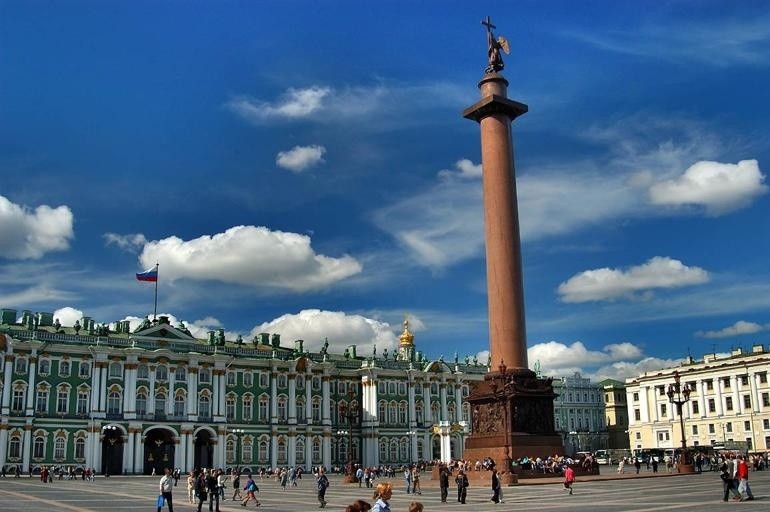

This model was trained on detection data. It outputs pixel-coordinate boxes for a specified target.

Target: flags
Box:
[136,261,159,282]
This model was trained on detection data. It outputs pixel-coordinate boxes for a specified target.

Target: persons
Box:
[344,483,424,512]
[404,456,501,504]
[581,450,770,474]
[260,465,304,491]
[152,466,260,511]
[720,452,754,501]
[3,464,96,484]
[565,464,576,495]
[512,453,575,474]
[316,471,329,509]
[313,464,396,488]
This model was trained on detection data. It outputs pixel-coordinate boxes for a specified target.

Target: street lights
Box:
[404,430,418,467]
[334,428,350,470]
[719,422,729,450]
[98,422,121,478]
[624,429,634,454]
[745,408,759,455]
[568,430,580,458]
[666,368,695,475]
[229,428,248,470]
[488,356,523,488]
[338,385,365,485]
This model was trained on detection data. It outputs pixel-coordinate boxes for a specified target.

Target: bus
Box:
[577,446,727,465]
